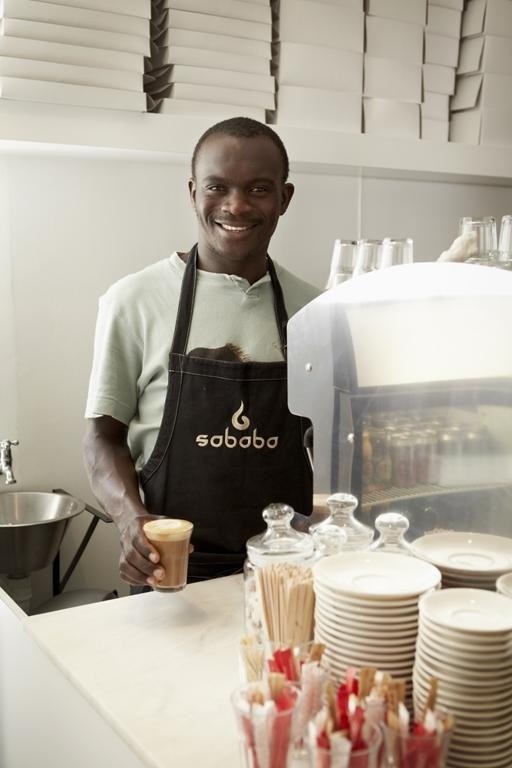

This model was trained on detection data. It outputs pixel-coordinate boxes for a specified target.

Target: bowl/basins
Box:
[0,492,87,577]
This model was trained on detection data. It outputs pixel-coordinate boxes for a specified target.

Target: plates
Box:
[313,533,512,742]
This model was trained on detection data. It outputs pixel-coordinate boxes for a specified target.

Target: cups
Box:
[326,235,413,294]
[141,517,194,594]
[458,213,512,272]
[232,677,452,767]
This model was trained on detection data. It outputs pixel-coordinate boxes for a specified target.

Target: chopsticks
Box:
[253,562,317,653]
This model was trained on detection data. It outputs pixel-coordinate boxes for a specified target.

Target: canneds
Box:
[360,405,493,493]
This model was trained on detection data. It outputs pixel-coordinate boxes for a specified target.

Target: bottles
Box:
[244,491,419,641]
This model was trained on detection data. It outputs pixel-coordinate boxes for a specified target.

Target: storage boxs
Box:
[1,0,511,151]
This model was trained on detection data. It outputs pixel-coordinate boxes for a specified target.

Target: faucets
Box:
[0,439,20,485]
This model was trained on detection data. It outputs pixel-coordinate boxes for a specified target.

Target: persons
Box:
[85,117,363,595]
